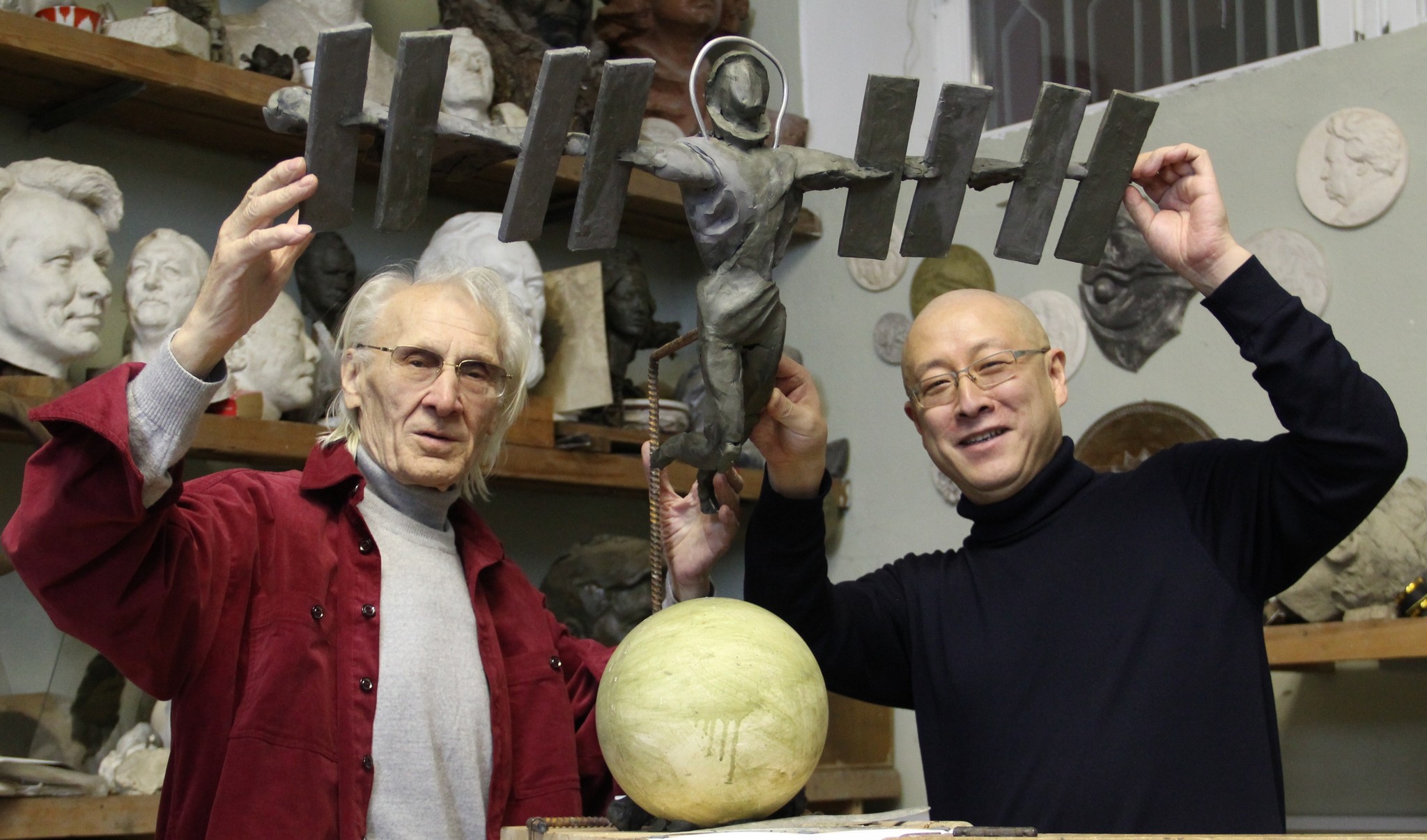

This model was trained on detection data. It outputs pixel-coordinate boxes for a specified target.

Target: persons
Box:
[597,0,811,146]
[221,289,318,425]
[267,49,1096,516]
[115,227,211,371]
[1,156,123,384]
[4,156,746,840]
[742,146,1410,834]
[538,533,668,648]
[293,229,358,341]
[1275,478,1427,625]
[578,268,680,428]
[441,26,495,126]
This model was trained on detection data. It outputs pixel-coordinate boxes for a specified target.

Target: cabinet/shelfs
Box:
[0,6,901,840]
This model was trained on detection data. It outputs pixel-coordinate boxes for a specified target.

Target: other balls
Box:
[595,596,831,827]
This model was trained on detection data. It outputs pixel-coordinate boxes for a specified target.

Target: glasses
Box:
[354,342,513,397]
[911,347,1047,407]
